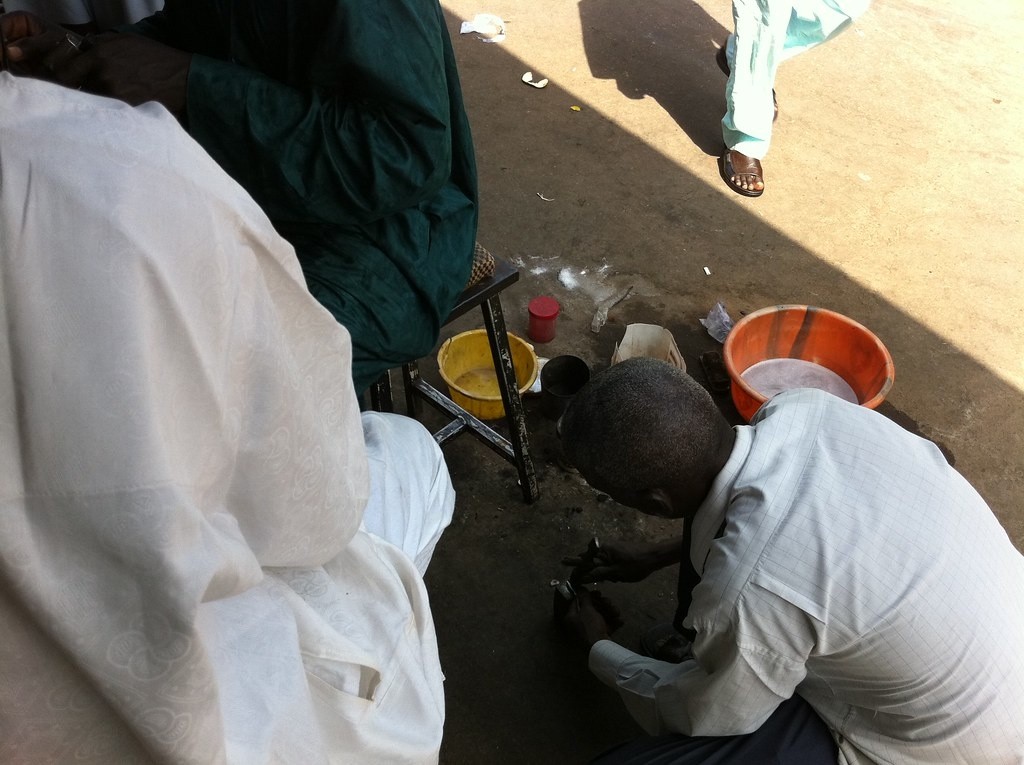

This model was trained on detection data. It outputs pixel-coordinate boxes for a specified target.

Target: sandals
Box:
[723,140,764,197]
[716,36,778,124]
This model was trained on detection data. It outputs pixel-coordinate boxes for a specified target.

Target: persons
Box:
[563,356,1024,765]
[0,64,458,763]
[716,1,871,197]
[0,0,479,397]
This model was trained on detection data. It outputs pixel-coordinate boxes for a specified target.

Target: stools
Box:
[362,242,541,502]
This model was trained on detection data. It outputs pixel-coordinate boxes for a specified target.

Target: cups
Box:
[541,354,590,422]
[527,298,559,344]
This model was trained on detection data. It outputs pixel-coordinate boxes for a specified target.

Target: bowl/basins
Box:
[723,303,895,424]
[436,328,538,420]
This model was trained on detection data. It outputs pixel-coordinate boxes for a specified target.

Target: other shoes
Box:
[642,623,695,664]
